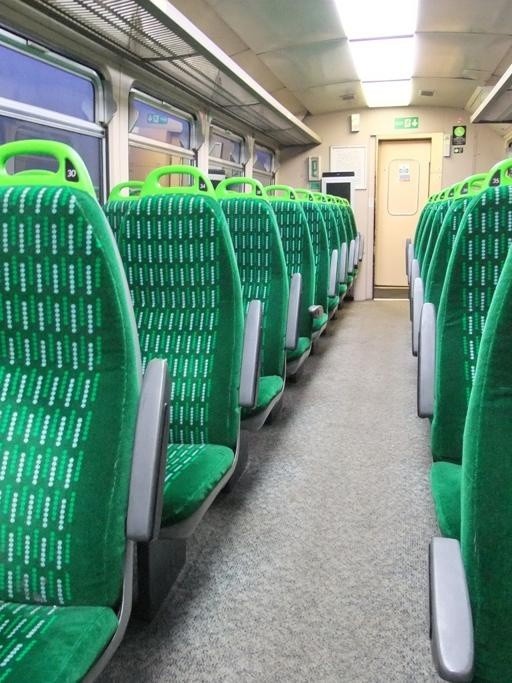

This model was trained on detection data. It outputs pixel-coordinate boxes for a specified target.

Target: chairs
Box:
[403,157,511,683]
[0,138,363,681]
